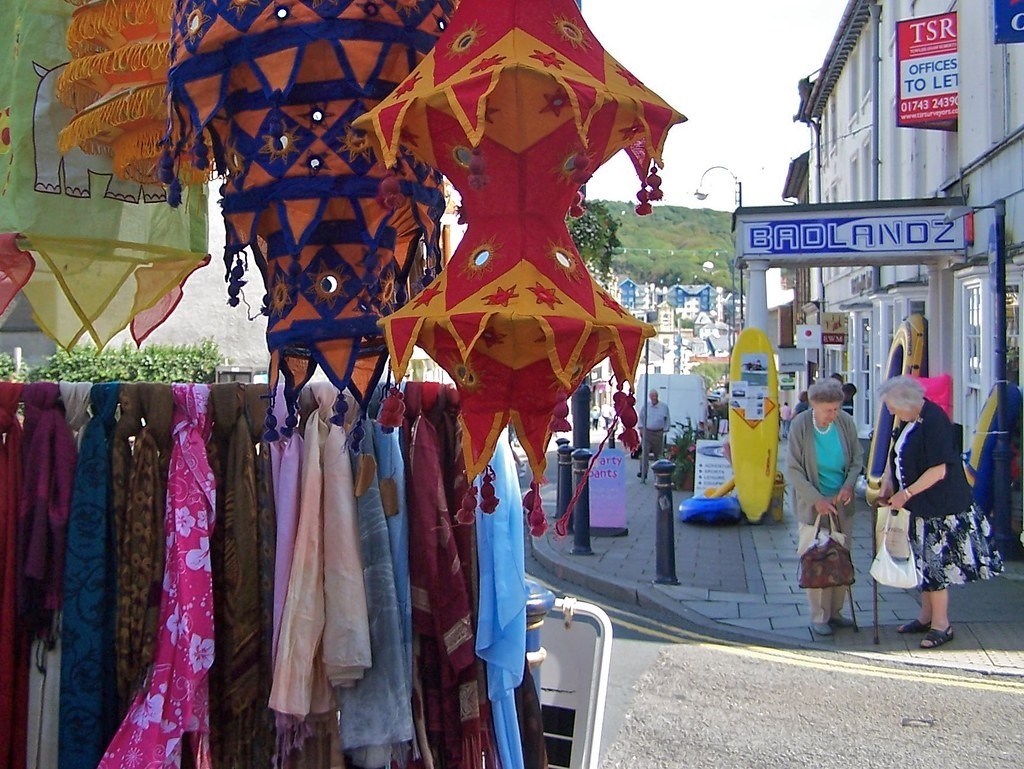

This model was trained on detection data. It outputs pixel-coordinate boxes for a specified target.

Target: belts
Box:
[646,428,663,432]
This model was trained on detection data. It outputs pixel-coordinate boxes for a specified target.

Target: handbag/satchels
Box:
[876,507,910,558]
[798,512,855,588]
[796,513,845,555]
[869,505,918,589]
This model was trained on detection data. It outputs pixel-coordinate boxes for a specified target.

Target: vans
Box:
[635,373,709,450]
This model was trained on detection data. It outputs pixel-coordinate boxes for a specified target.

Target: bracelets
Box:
[905,489,912,499]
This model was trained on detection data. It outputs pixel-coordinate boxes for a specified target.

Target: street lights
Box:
[702,246,735,348]
[943,198,1024,561]
[693,166,745,335]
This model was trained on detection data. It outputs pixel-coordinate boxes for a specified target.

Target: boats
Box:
[677,487,742,524]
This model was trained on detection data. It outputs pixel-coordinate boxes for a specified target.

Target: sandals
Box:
[920,626,953,648]
[898,618,931,633]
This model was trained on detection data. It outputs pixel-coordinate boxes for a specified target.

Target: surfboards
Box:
[726,327,780,527]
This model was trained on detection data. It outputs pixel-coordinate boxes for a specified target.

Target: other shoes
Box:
[812,623,833,636]
[833,616,852,625]
[637,473,647,478]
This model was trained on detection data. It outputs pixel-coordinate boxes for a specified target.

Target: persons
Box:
[786,379,863,634]
[715,383,729,431]
[778,403,791,441]
[637,389,670,478]
[877,376,1004,648]
[792,391,809,417]
[830,373,857,416]
[590,402,613,429]
